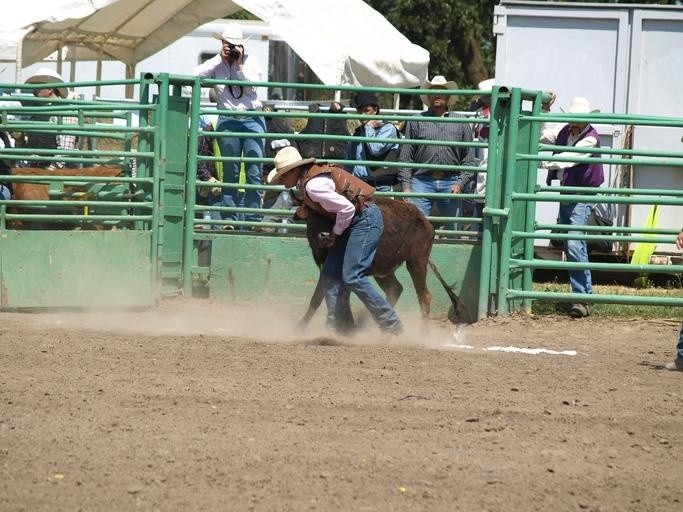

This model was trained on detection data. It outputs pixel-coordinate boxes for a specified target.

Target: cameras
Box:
[229,43,240,61]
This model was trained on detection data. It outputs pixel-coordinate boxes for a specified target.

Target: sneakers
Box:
[569,299,591,318]
[224,224,235,231]
[251,224,264,232]
[666,361,682,372]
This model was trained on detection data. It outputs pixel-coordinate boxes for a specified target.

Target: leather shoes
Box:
[380,322,403,344]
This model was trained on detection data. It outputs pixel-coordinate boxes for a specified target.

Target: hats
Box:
[349,93,379,108]
[421,73,459,107]
[473,77,496,101]
[267,146,316,184]
[559,96,601,114]
[22,66,70,99]
[210,24,253,46]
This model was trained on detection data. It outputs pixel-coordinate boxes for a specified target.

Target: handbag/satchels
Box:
[587,214,612,254]
[365,121,404,185]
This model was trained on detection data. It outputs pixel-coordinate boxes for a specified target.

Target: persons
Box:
[539,96,604,316]
[266,146,406,340]
[666,227,683,371]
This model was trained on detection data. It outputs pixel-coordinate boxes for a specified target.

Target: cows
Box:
[289,188,472,336]
[7,165,132,230]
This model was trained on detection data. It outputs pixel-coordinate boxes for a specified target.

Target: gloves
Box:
[200,186,209,198]
[208,177,222,195]
